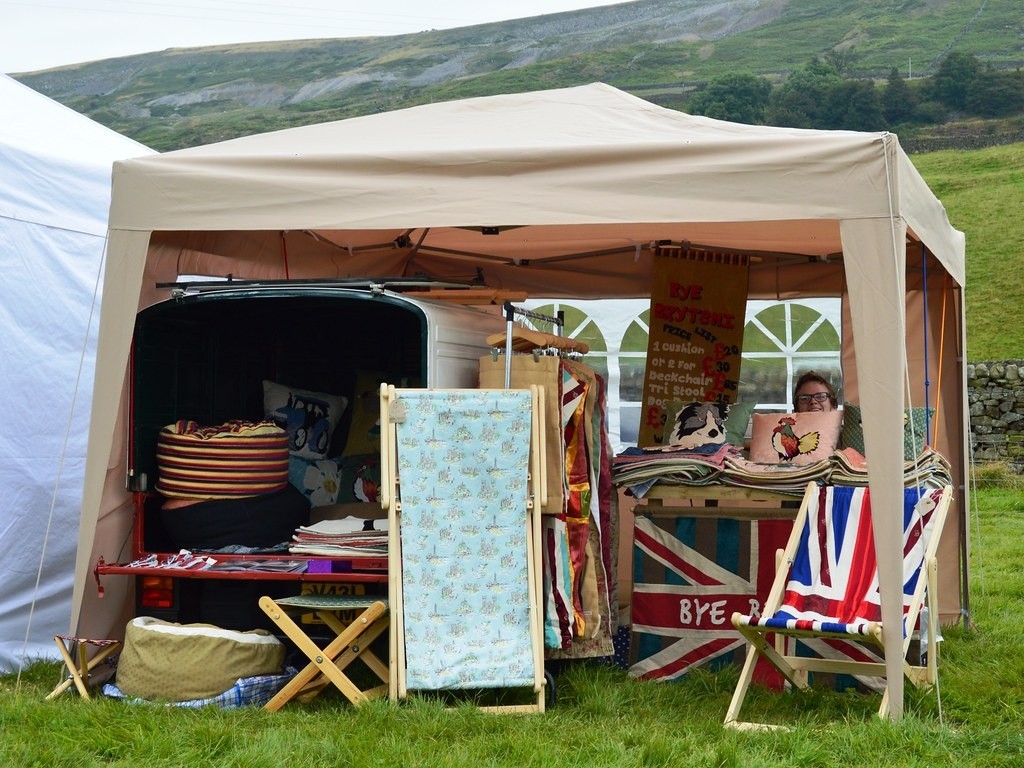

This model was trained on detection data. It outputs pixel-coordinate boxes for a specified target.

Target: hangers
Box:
[487,307,589,362]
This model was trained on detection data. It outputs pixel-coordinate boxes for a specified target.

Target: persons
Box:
[792,370,839,412]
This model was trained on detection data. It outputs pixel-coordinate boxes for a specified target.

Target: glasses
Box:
[795,392,831,404]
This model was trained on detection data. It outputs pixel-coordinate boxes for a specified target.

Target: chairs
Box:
[722,481,955,733]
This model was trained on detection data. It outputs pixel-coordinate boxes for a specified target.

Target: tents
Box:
[0,70,159,676]
[63,81,971,728]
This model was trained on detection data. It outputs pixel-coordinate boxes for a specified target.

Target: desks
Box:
[623,483,804,678]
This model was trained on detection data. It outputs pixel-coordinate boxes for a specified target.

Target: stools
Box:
[258,594,389,714]
[45,633,121,703]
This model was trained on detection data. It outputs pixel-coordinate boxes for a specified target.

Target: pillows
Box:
[842,401,936,461]
[749,409,845,466]
[662,399,759,454]
[262,379,386,505]
